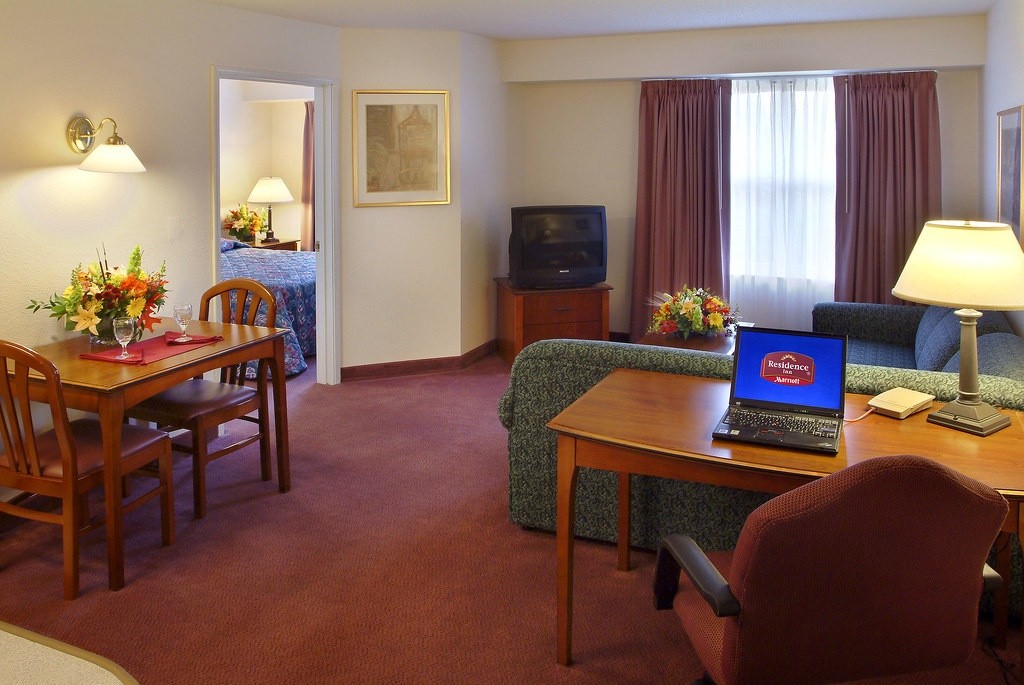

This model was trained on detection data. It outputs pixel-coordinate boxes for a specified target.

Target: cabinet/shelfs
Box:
[493,277,615,367]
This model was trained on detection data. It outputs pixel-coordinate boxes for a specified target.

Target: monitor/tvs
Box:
[508,205,607,287]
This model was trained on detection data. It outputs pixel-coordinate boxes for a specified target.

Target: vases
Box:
[678,330,707,338]
[89,315,138,348]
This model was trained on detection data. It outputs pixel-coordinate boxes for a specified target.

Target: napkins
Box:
[78,331,225,367]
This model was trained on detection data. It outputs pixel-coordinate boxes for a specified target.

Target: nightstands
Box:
[244,238,301,251]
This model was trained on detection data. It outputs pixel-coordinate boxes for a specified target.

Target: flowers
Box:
[643,280,743,339]
[25,241,172,340]
[223,203,268,236]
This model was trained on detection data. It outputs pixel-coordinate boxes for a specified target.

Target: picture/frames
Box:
[996,104,1024,253]
[351,88,452,208]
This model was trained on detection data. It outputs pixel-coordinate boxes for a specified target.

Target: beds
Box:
[221,237,317,380]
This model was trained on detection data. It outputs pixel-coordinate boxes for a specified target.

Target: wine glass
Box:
[113,317,135,359]
[174,304,193,342]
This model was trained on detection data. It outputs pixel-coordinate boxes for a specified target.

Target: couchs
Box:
[812,302,1024,382]
[498,338,1024,627]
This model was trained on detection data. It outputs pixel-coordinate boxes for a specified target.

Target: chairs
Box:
[0,339,176,600]
[649,455,1011,685]
[122,277,278,518]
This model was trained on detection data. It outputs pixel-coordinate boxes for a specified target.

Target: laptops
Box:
[712,325,848,454]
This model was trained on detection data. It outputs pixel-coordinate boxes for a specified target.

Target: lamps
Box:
[892,220,1024,438]
[247,176,294,243]
[65,116,147,174]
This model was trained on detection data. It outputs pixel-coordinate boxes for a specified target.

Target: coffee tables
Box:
[636,322,755,356]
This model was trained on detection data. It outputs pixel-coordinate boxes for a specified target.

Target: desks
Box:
[547,368,1024,668]
[0,316,293,590]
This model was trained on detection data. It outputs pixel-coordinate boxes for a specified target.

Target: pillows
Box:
[220,238,251,253]
